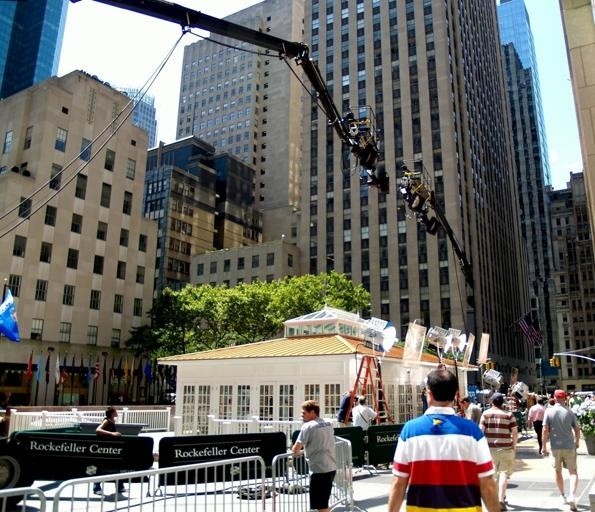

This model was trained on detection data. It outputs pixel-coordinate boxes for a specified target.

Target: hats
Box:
[553,390,566,400]
[490,392,504,406]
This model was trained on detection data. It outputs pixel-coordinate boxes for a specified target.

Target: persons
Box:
[335,388,358,427]
[387,368,503,512]
[93,406,131,496]
[460,388,595,511]
[292,399,336,512]
[351,396,377,452]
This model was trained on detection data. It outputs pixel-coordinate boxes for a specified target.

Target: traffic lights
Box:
[487,362,492,370]
[550,359,554,367]
[555,359,560,366]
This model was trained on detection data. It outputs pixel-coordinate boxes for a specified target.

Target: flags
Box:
[518,312,544,347]
[0,288,21,342]
[24,351,176,386]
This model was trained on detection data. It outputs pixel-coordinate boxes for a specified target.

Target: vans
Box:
[570,391,594,401]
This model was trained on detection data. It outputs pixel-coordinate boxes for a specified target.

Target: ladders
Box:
[344,355,395,426]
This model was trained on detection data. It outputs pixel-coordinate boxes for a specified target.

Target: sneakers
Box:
[499,502,507,511]
[559,494,578,511]
